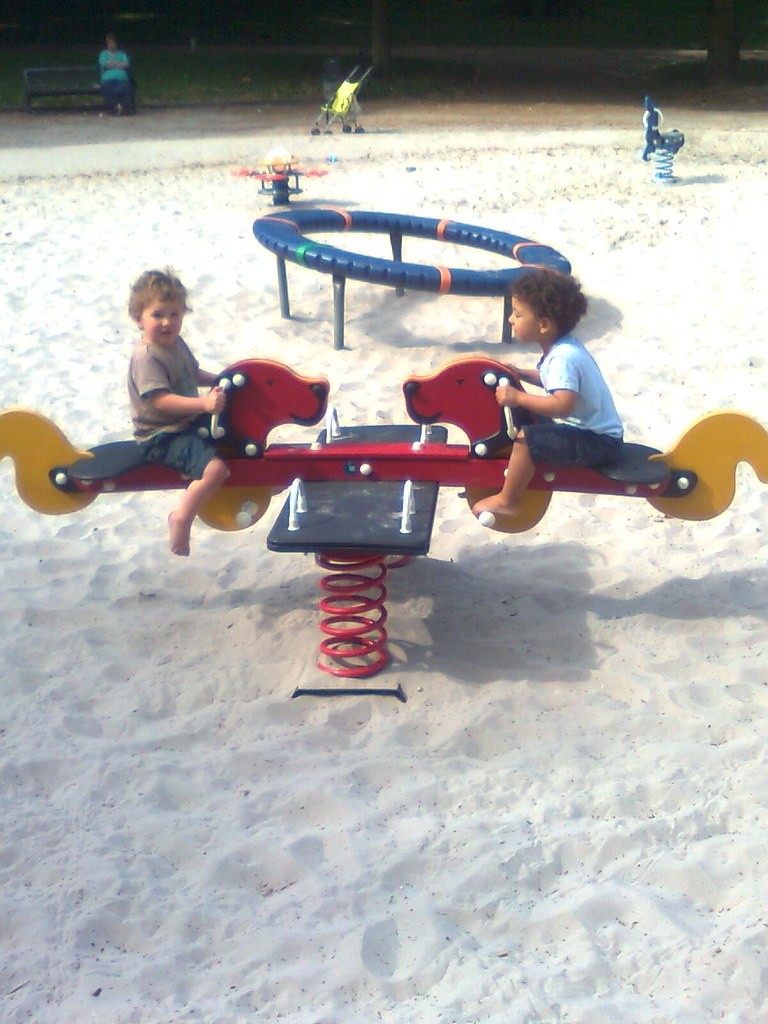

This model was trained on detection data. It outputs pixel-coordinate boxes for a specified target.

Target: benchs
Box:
[23,66,138,114]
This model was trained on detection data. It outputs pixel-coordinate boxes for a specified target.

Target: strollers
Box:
[311,65,370,136]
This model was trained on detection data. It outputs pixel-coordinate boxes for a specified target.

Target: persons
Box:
[98,32,132,114]
[472,269,625,520]
[128,269,230,556]
